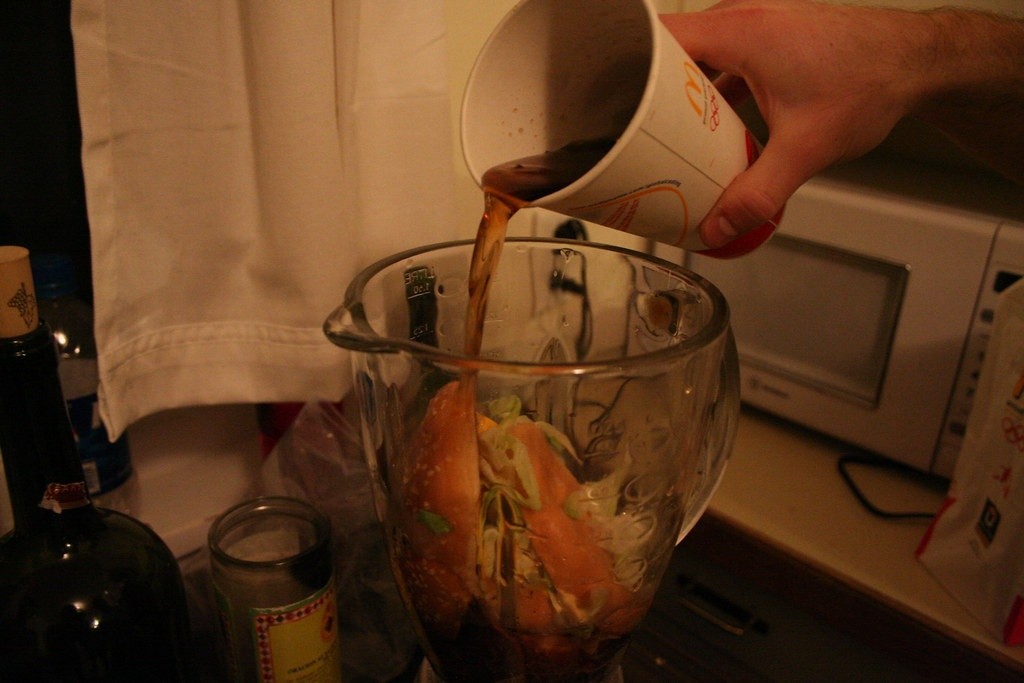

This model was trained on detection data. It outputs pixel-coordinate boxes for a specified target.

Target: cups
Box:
[459,0,791,260]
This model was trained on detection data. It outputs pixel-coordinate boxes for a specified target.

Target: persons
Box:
[657,0,1024,248]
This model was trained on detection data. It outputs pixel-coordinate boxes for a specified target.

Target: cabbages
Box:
[417,396,657,623]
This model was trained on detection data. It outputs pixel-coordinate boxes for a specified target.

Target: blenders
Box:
[320,237,741,683]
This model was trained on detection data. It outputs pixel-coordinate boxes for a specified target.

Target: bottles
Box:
[0,243,203,683]
[29,267,143,517]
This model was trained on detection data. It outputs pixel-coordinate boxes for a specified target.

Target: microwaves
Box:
[642,176,1023,481]
